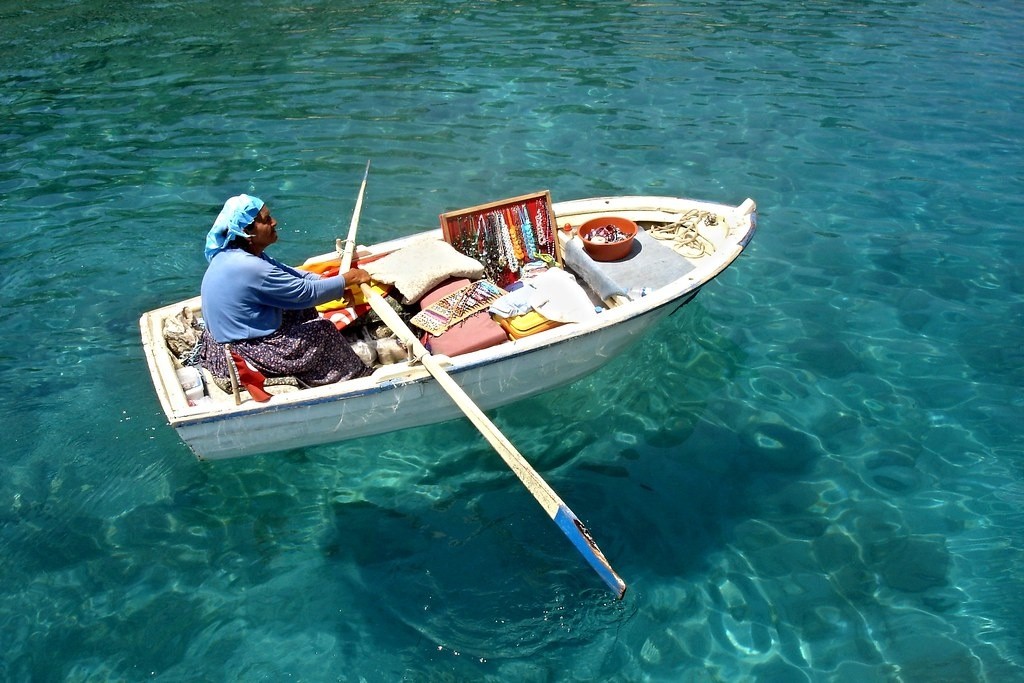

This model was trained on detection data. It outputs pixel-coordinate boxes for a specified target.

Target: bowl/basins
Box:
[578,216,638,260]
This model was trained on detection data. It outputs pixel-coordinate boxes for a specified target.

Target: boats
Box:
[140,195,755,462]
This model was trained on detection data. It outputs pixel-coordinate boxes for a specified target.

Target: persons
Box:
[196,193,377,390]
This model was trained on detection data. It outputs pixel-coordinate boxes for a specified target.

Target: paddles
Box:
[337,158,372,277]
[358,278,631,601]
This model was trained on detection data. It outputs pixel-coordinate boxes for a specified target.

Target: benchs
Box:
[199,365,297,407]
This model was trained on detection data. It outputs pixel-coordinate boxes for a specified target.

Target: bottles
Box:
[563,223,573,239]
